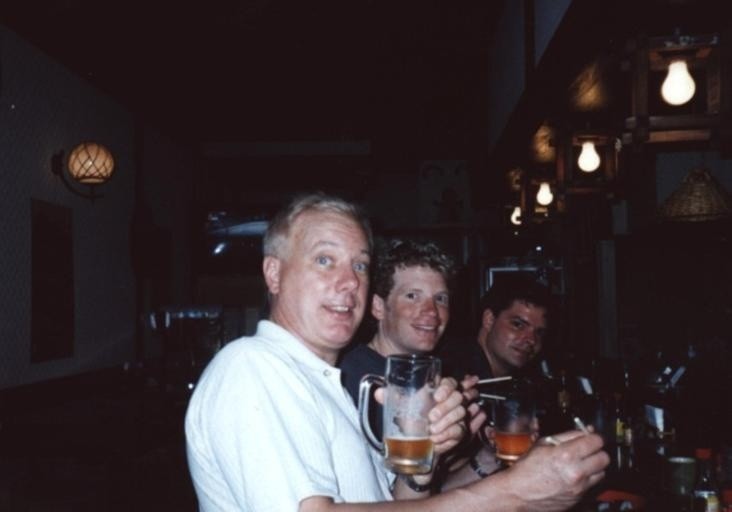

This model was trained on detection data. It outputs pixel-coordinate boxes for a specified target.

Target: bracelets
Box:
[400,472,431,492]
[469,458,488,479]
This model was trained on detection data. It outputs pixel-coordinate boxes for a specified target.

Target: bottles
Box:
[686,447,723,512]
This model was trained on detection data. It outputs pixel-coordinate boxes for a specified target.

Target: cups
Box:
[666,457,697,496]
[356,352,443,475]
[476,377,543,462]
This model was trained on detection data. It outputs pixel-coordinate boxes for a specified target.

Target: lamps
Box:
[577,139,602,172]
[656,49,696,107]
[535,181,554,206]
[52,139,116,206]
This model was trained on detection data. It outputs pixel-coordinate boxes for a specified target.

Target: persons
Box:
[340,235,541,494]
[183,192,612,512]
[447,270,551,451]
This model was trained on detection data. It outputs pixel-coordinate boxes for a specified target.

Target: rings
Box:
[458,422,467,436]
